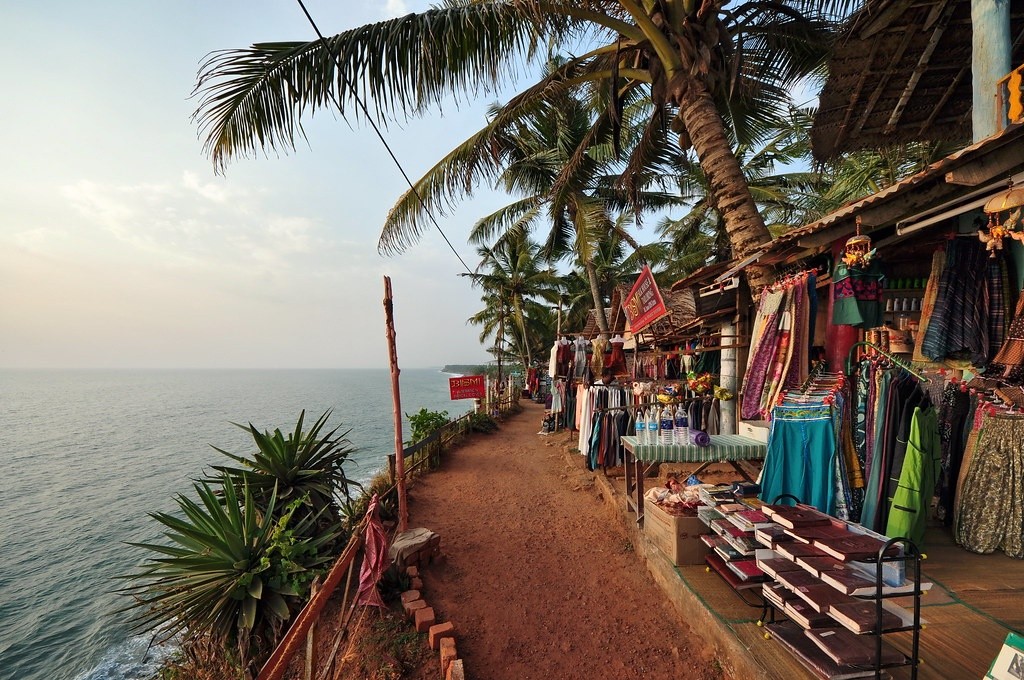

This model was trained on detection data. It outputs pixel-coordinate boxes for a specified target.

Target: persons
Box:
[590,335,608,374]
[594,368,616,384]
[608,334,627,374]
[573,336,590,377]
[555,337,572,376]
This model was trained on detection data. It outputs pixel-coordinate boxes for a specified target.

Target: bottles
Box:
[660,407,673,445]
[635,412,645,444]
[648,412,658,445]
[675,407,689,446]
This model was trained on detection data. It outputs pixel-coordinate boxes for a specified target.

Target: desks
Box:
[620,433,769,528]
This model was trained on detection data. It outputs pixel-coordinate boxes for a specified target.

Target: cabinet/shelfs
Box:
[696,479,801,627]
[754,495,929,680]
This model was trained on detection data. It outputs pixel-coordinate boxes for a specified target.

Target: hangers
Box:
[595,394,720,416]
[761,259,819,290]
[778,342,1024,414]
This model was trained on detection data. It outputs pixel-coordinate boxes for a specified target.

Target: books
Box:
[697,481,906,680]
